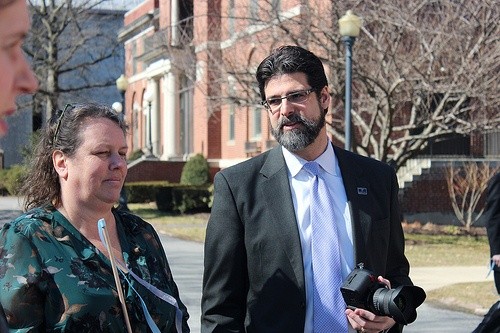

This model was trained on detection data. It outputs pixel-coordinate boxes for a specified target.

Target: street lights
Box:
[144,90,156,159]
[337,9,363,152]
[116,74,129,159]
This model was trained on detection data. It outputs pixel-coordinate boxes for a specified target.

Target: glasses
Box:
[52,103,84,151]
[260,87,320,111]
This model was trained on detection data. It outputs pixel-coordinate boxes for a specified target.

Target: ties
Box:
[302,162,349,333]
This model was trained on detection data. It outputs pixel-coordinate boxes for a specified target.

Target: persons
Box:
[199,45,415,333]
[0,102,191,333]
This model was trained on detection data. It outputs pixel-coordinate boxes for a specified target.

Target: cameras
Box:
[340,262,426,326]
